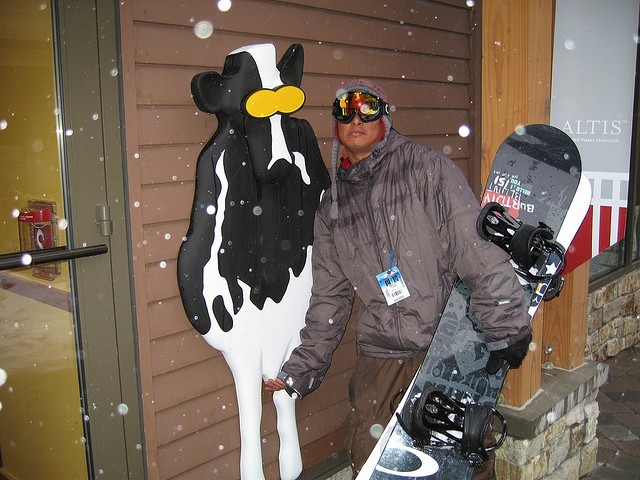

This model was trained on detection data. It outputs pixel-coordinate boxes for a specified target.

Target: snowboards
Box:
[368,123,582,480]
[353,171,592,480]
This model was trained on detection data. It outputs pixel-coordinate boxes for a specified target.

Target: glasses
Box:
[331,91,388,124]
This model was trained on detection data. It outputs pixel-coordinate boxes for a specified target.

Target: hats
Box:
[328,77,394,220]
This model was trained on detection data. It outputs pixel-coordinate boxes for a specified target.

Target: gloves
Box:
[484,328,533,376]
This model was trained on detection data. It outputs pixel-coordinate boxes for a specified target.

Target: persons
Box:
[265,78,534,480]
